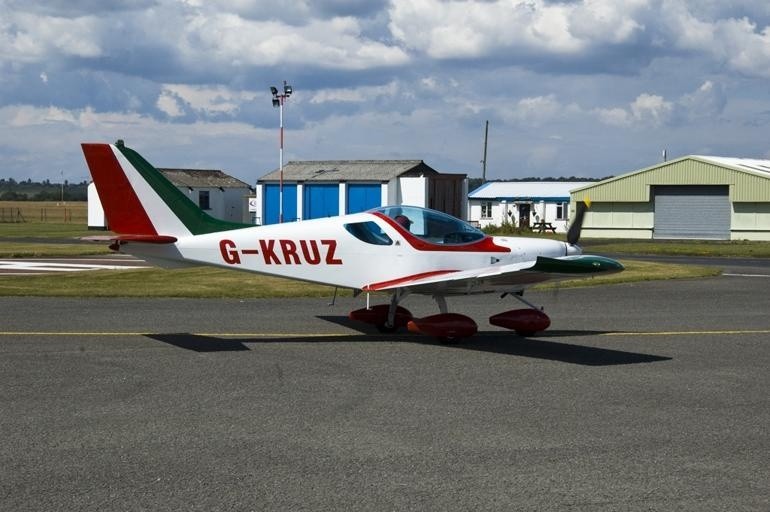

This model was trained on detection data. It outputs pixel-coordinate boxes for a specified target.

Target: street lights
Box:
[268,81,293,225]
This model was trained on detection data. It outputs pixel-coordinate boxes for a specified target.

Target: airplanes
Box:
[80,135,626,344]
